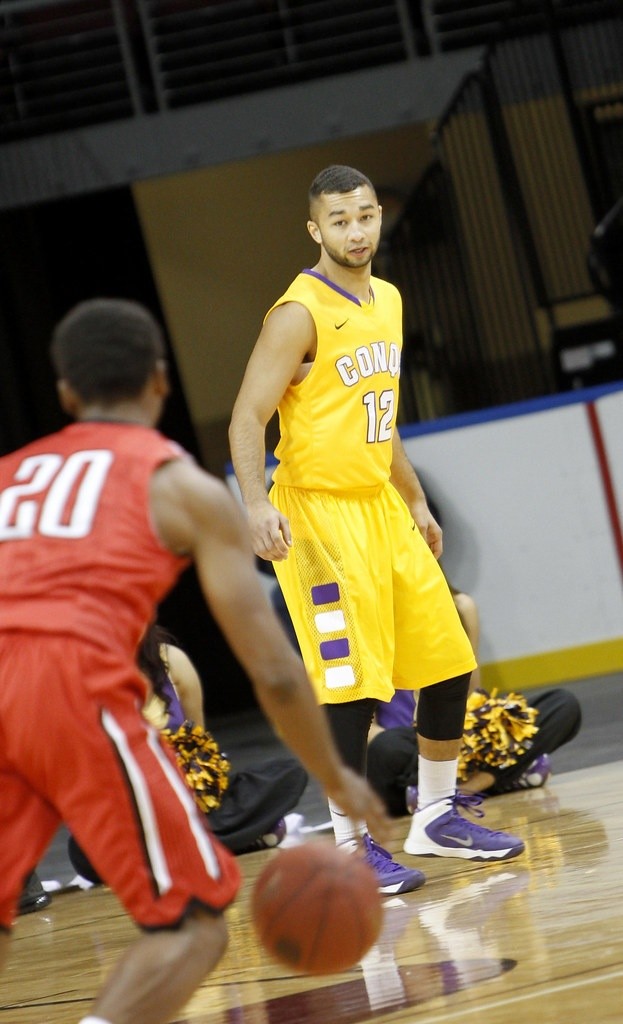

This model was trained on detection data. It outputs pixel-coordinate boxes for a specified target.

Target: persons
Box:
[18,619,310,919]
[227,166,525,895]
[254,446,581,820]
[0,302,399,1024]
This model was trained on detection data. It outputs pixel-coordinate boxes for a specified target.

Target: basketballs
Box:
[250,841,383,973]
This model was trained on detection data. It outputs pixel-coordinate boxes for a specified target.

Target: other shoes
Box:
[19,892,50,917]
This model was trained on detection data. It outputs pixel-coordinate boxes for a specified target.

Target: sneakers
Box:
[487,753,551,795]
[403,789,527,862]
[236,818,287,854]
[406,786,418,814]
[342,833,425,895]
